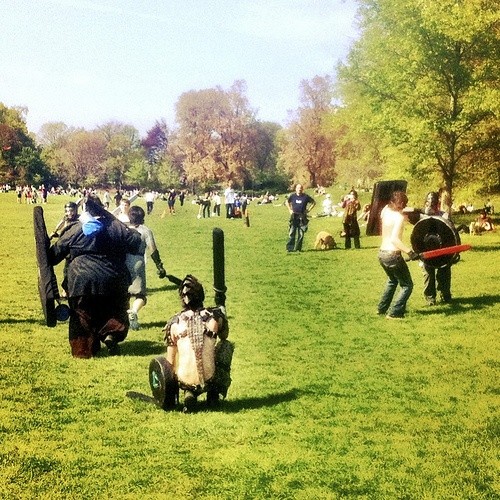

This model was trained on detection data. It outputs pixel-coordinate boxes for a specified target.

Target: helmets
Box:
[64,201,77,208]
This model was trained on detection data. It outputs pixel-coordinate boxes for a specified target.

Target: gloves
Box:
[150,249,166,279]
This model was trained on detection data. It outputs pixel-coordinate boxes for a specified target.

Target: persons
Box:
[341,189,361,250]
[0,184,376,227]
[409,199,494,236]
[49,201,83,241]
[375,191,420,320]
[163,274,234,411]
[285,184,316,253]
[49,194,142,358]
[125,205,166,331]
[406,191,451,305]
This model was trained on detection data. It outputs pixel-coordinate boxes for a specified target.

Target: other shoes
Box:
[441,295,451,302]
[184,392,197,412]
[386,313,406,320]
[428,299,436,306]
[104,335,120,349]
[128,311,139,331]
[376,309,387,316]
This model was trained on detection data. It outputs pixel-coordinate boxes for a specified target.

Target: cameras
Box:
[348,195,354,199]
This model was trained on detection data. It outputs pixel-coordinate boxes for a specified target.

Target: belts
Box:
[380,249,401,255]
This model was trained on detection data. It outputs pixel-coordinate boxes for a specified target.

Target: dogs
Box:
[314,231,336,250]
